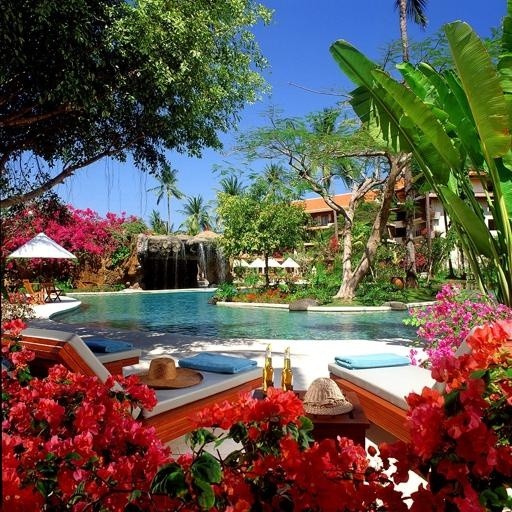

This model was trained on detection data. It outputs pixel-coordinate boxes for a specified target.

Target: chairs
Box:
[327,324,492,452]
[23,278,62,304]
[5,324,264,450]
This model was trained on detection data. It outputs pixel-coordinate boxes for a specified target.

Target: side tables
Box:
[249,388,371,453]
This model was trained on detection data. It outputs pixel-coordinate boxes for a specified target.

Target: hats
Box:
[302,378,353,415]
[124,358,203,389]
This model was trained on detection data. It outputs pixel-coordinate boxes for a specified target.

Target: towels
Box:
[334,351,410,371]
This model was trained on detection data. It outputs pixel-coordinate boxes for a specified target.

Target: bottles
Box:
[281,347,293,393]
[262,344,276,395]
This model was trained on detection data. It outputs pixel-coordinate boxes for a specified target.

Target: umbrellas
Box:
[9,233,76,283]
